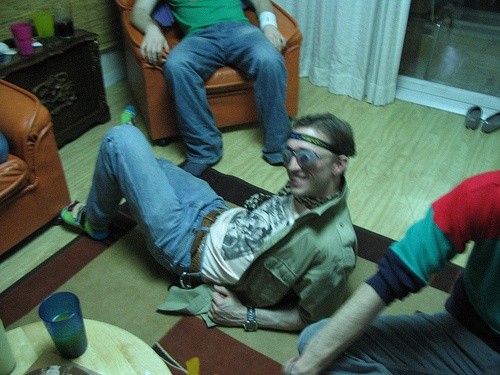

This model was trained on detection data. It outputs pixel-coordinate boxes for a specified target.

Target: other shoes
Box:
[465,104,500,133]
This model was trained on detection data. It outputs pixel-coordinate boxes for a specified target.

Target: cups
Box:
[38,292,88,359]
[32,11,54,39]
[10,22,33,54]
[0,321,16,375]
[55,5,75,39]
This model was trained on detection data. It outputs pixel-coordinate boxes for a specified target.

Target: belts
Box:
[190,209,226,284]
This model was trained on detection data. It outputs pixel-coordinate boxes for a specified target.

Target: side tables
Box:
[0,28,111,150]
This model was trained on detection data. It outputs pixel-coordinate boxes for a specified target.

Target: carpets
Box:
[152,311,286,375]
[0,158,466,375]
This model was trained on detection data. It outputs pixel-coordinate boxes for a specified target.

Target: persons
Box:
[59,102,359,333]
[128,1,295,178]
[281,166,500,375]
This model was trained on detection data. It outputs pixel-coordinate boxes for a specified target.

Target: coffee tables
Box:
[5,318,171,375]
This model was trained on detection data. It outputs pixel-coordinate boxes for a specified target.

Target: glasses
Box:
[282,147,338,167]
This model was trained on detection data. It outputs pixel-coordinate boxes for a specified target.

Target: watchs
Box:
[242,305,258,332]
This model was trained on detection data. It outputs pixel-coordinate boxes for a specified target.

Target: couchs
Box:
[0,78,72,257]
[115,0,304,147]
